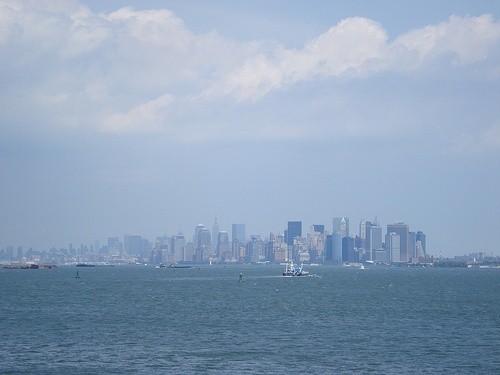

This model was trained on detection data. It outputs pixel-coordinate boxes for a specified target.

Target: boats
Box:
[343,262,365,270]
[282,267,310,276]
[3,261,59,270]
[154,263,193,269]
[75,263,115,267]
[480,265,500,268]
